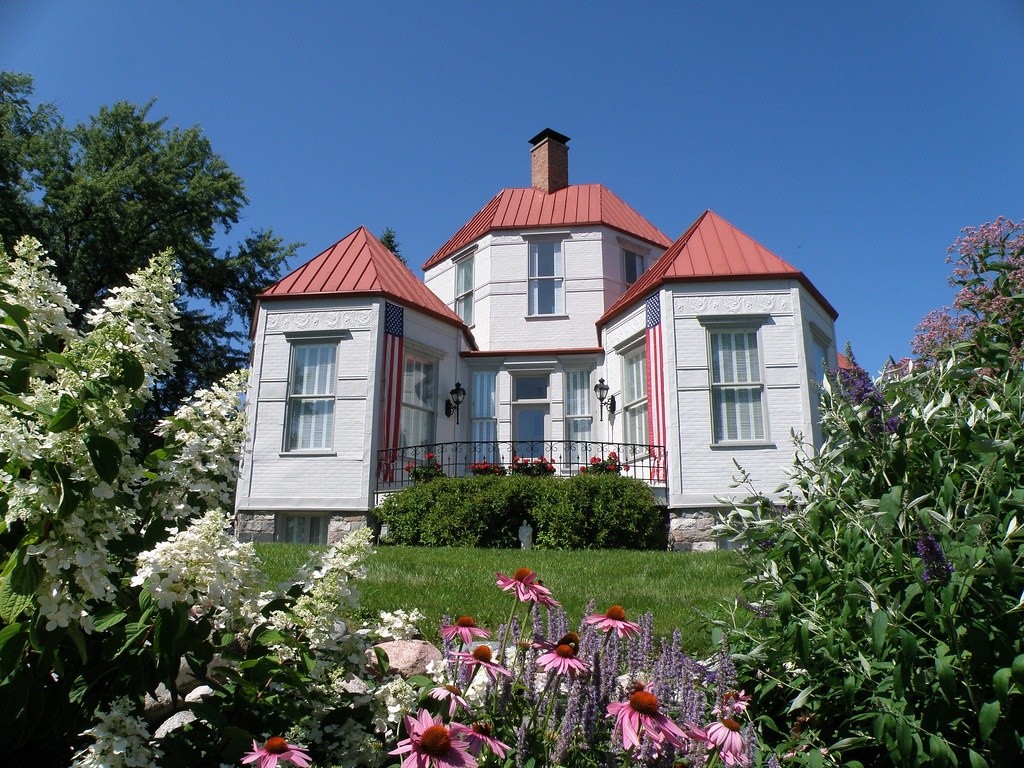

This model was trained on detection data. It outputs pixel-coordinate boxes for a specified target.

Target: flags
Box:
[645,291,667,482]
[382,300,404,482]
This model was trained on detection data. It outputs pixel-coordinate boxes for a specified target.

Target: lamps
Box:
[445,380,466,425]
[593,378,615,422]
